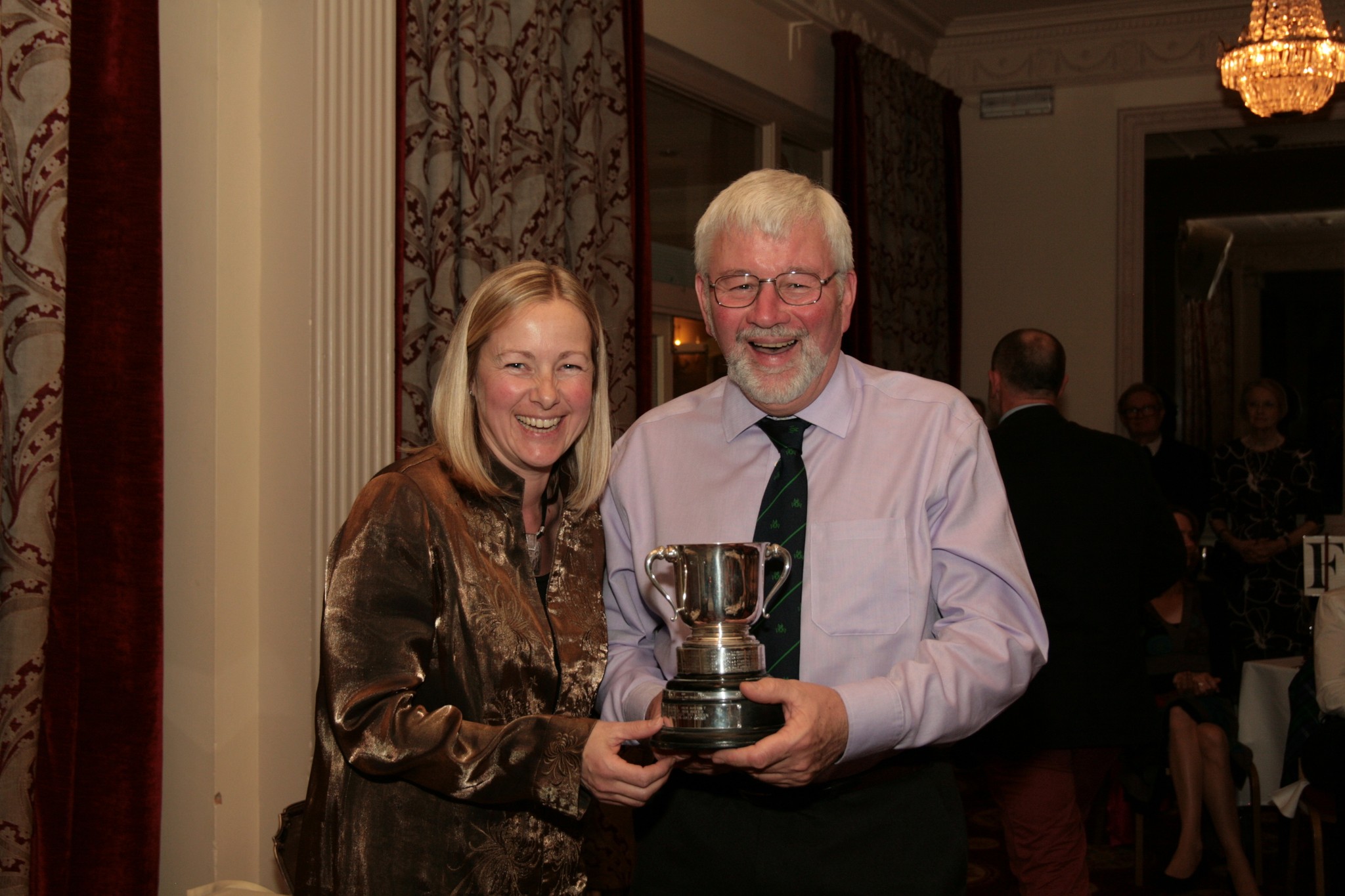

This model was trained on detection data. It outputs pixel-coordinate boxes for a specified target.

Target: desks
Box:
[1232,654,1312,812]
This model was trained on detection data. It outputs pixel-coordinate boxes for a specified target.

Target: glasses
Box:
[708,267,840,308]
[1121,404,1162,418]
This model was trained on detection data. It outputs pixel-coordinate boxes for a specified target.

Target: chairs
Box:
[1285,750,1345,896]
[1113,725,1267,888]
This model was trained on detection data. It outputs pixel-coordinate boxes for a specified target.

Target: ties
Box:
[753,416,813,726]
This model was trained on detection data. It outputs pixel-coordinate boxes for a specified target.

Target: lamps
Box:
[1213,1,1345,118]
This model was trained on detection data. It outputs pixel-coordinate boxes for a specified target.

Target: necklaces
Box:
[523,490,548,573]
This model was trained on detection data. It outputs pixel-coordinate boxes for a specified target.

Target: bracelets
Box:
[1217,527,1229,538]
[1282,533,1293,550]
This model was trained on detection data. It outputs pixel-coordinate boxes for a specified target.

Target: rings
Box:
[1198,682,1203,686]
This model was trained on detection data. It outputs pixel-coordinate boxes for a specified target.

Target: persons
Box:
[602,168,1054,895]
[294,258,679,895]
[987,328,1184,896]
[966,396,998,444]
[1136,507,1266,896]
[1118,381,1208,534]
[1202,376,1329,796]
